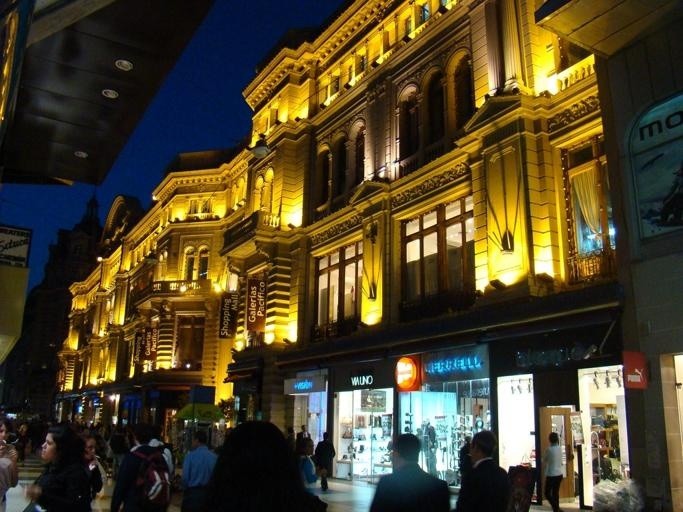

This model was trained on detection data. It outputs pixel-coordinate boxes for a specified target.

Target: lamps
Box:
[590,370,621,389]
[506,377,532,395]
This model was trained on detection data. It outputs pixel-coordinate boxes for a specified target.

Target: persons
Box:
[456,429,510,511]
[369,434,451,512]
[1,416,336,511]
[541,431,567,512]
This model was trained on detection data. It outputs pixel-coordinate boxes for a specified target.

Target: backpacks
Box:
[132,447,172,510]
